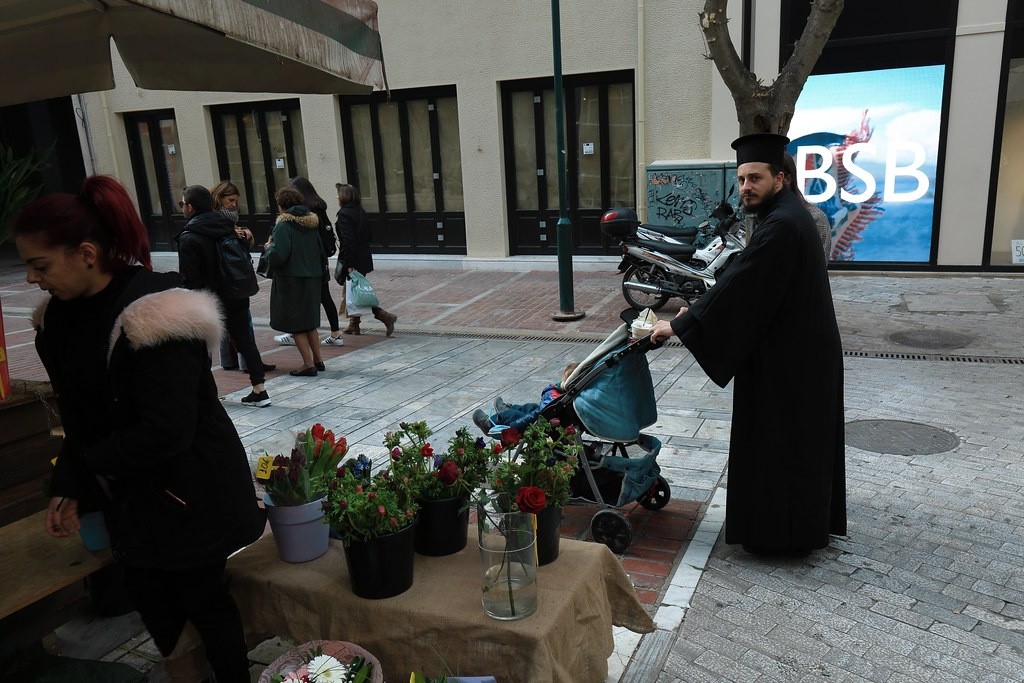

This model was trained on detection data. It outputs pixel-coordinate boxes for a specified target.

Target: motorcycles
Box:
[600,182,747,311]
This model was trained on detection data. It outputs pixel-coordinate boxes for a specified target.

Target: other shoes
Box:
[290,366,317,376]
[244,363,276,373]
[314,362,325,371]
[223,364,240,370]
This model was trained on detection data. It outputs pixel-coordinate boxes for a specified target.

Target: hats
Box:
[731,134,790,168]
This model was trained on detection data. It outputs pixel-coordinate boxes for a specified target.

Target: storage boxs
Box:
[600,208,640,237]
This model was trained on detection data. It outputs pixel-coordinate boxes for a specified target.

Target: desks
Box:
[161,529,655,683]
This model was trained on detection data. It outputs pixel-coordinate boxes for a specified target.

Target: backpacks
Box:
[211,233,259,299]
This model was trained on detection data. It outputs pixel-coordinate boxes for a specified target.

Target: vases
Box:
[477,531,539,621]
[340,524,417,599]
[502,501,562,567]
[259,638,384,683]
[411,490,471,557]
[263,493,333,563]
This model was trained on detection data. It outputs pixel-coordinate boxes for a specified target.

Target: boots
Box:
[374,308,397,336]
[343,316,360,335]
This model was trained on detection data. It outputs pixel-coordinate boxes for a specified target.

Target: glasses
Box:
[179,201,188,208]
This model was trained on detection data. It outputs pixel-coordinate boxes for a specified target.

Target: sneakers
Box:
[494,395,512,413]
[241,390,271,407]
[321,334,344,346]
[473,408,494,437]
[274,332,297,345]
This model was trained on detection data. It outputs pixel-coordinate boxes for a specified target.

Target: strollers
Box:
[485,305,672,552]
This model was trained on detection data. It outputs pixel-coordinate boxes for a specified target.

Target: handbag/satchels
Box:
[345,277,372,319]
[334,262,348,286]
[323,216,337,257]
[257,247,273,279]
[349,270,379,307]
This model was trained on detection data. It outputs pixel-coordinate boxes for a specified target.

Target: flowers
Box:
[263,409,584,540]
[271,646,371,683]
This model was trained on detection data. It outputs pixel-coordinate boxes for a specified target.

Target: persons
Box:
[210,181,276,372]
[274,177,343,346]
[173,185,271,407]
[7,176,269,683]
[264,186,325,377]
[335,185,397,337]
[649,133,846,559]
[473,362,580,440]
[745,153,831,265]
